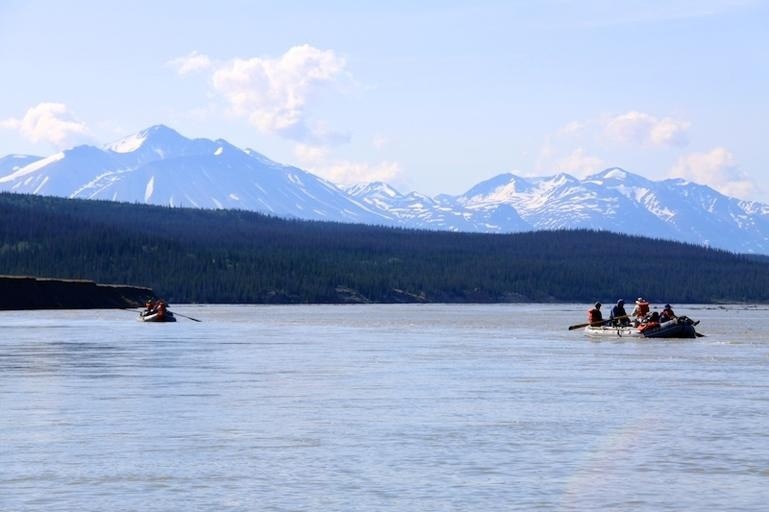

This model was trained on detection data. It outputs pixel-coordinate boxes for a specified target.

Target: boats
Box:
[585,317,697,339]
[135,311,177,322]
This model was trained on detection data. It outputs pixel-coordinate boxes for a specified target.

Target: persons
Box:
[587,301,603,327]
[606,299,633,327]
[631,297,678,328]
[145,296,166,317]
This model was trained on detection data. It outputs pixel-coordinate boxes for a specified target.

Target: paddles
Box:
[569,314,632,330]
[172,312,201,322]
